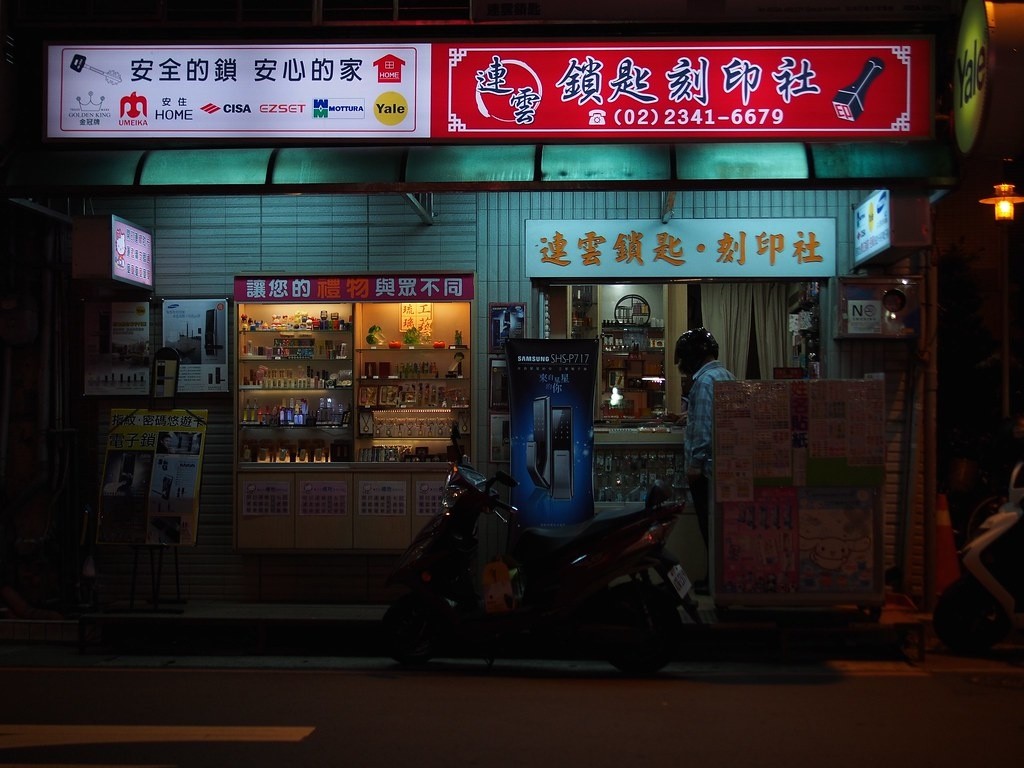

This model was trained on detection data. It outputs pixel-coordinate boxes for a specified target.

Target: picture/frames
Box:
[488,355,510,410]
[487,300,528,357]
[488,413,510,464]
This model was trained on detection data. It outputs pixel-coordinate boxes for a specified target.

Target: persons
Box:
[662,329,737,598]
[159,433,175,454]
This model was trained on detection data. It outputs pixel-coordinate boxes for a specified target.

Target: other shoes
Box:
[694,578,712,595]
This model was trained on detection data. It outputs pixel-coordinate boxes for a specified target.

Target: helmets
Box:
[675,327,719,381]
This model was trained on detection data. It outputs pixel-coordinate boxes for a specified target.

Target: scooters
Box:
[932,459,1024,655]
[380,422,709,678]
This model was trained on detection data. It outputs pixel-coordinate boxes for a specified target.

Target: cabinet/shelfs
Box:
[355,300,474,551]
[234,301,355,548]
